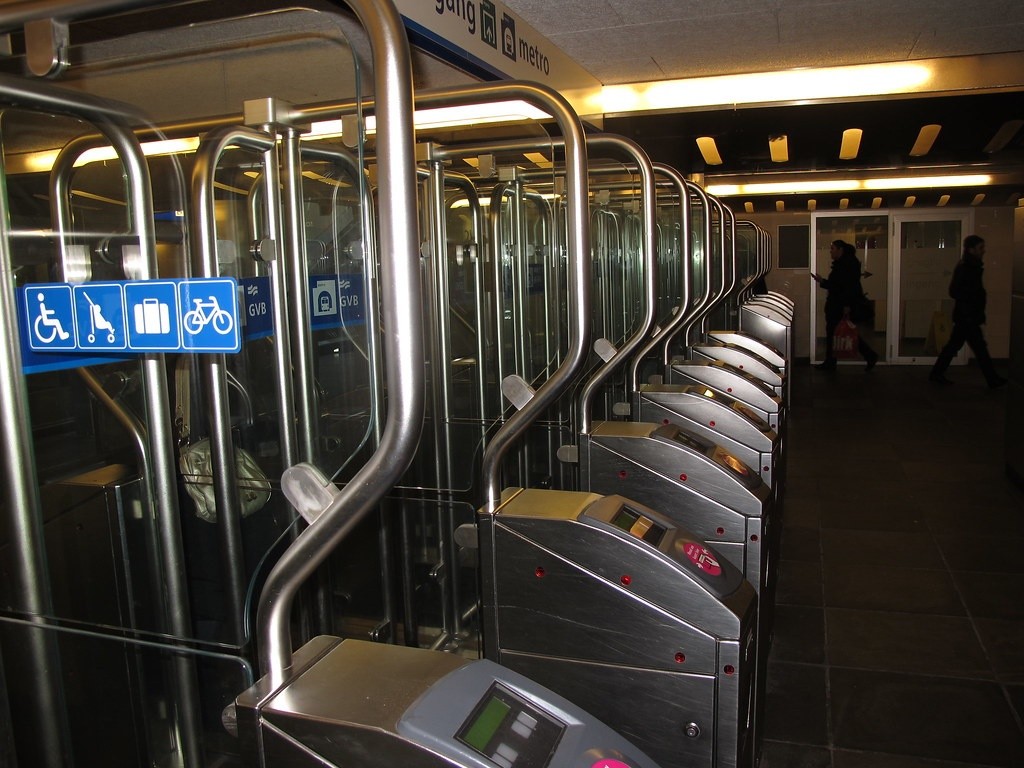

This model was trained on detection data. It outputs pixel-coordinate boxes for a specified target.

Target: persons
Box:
[809,240,878,373]
[928,234,1009,390]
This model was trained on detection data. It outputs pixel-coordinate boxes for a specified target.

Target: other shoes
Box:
[864,351,879,372]
[928,368,952,383]
[989,377,1009,389]
[813,358,836,370]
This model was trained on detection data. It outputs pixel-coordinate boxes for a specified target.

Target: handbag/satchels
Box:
[832,309,858,355]
